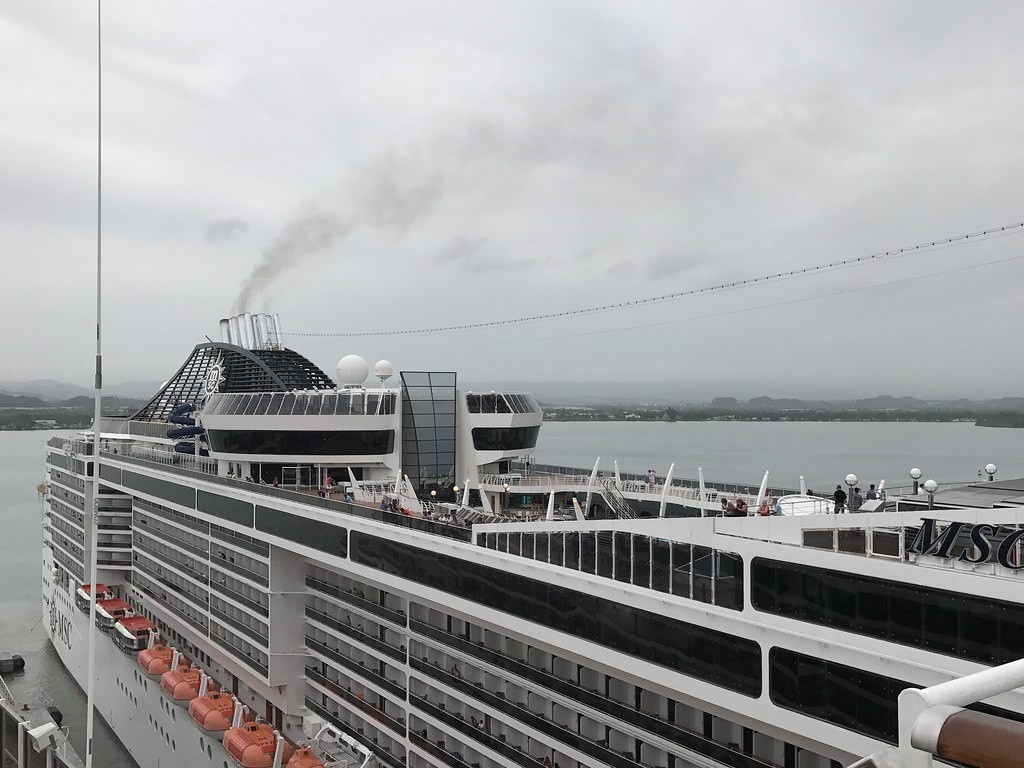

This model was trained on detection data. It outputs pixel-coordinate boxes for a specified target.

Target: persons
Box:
[380,500,476,541]
[917,483,925,496]
[720,483,878,517]
[345,615,352,627]
[470,716,483,732]
[318,474,355,516]
[351,588,365,599]
[543,756,553,767]
[218,550,234,564]
[646,469,657,489]
[220,577,226,586]
[450,663,461,678]
[226,472,279,496]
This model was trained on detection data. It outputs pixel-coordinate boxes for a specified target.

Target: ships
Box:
[38,311,1023,768]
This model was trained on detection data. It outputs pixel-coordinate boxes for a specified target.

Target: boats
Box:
[75,583,326,768]
